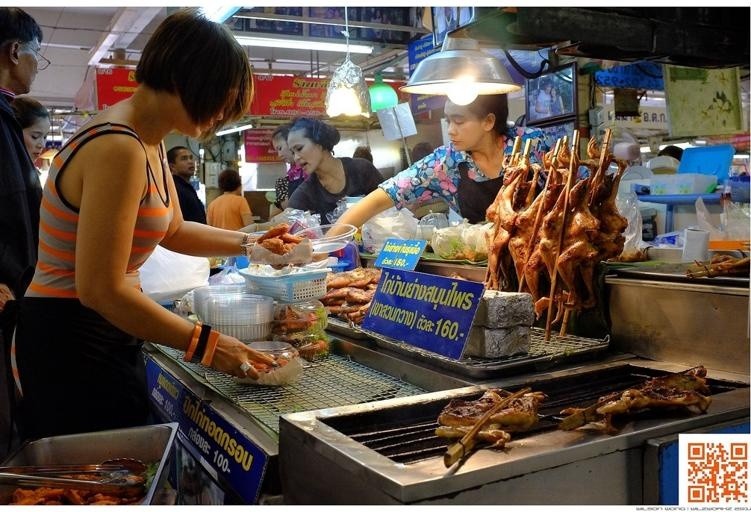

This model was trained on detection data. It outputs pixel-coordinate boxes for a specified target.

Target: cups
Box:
[16,38,51,74]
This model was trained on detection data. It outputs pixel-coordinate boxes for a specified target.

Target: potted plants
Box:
[524,62,578,126]
[534,117,578,150]
[660,64,746,138]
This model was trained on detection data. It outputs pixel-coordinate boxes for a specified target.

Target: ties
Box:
[525,60,579,128]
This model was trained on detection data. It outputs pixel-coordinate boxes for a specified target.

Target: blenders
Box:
[318,265,382,325]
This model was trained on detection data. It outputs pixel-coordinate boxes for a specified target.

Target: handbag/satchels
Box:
[239,233,257,258]
[180,321,222,367]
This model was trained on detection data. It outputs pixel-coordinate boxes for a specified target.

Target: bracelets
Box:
[187,286,298,376]
[288,224,358,254]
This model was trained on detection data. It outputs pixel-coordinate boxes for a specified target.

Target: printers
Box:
[0,458,147,496]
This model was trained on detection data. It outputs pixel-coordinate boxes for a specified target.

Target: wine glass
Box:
[239,359,252,374]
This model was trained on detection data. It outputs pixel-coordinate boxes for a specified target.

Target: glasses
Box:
[324,7,371,119]
[398,8,522,107]
[369,69,399,111]
[39,116,59,159]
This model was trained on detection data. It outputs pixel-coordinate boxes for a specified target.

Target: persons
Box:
[204,169,257,231]
[269,125,308,220]
[314,91,591,244]
[549,88,565,115]
[352,146,375,164]
[12,88,53,165]
[535,80,554,118]
[412,142,435,163]
[2,10,313,439]
[285,117,388,229]
[1,7,47,442]
[166,144,209,225]
